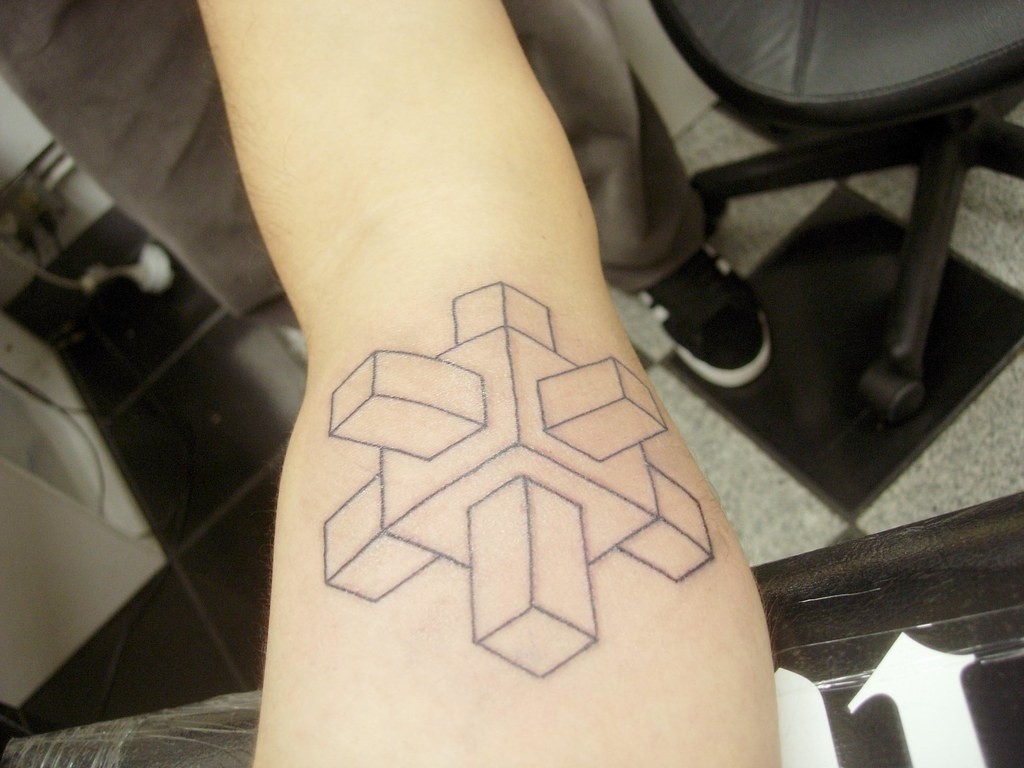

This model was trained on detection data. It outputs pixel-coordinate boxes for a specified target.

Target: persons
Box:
[0,1,772,768]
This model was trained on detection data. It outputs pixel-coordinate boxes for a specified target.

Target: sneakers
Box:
[635,240,770,387]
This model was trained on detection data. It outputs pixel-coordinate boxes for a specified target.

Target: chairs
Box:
[651,0,1024,426]
